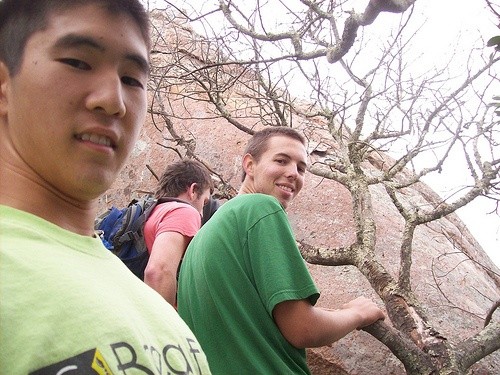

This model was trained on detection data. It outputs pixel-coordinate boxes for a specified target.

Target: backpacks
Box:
[93,195,194,281]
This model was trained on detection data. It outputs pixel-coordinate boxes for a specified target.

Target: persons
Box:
[0,0,212,375]
[141,124,387,375]
